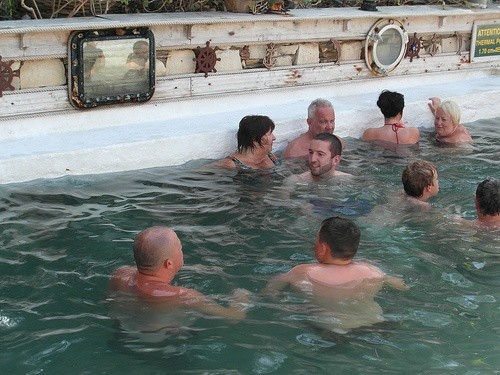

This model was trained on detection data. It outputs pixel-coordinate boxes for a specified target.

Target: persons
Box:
[133,227,187,296]
[402,160,438,203]
[215,115,277,169]
[428,97,470,142]
[474,179,500,222]
[300,132,349,179]
[123,42,148,92]
[83,41,107,93]
[295,216,384,283]
[284,98,345,157]
[361,89,419,144]
